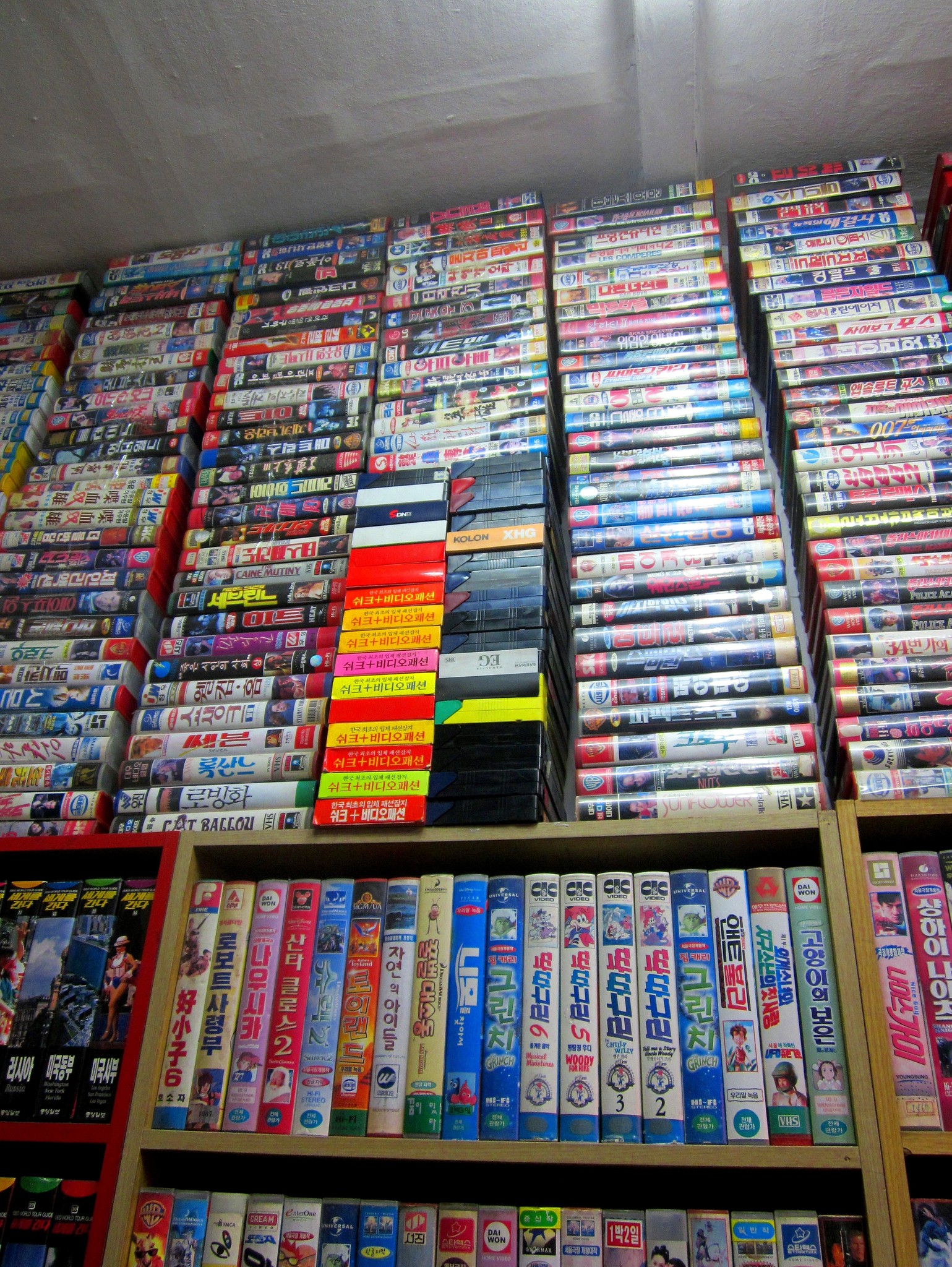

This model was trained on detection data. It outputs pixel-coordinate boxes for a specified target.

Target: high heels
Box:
[109,1033,120,1043]
[101,1031,111,1040]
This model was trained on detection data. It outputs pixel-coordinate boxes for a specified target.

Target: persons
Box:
[651,1245,686,1267]
[876,892,906,935]
[82,590,124,615]
[255,272,285,286]
[827,1229,866,1267]
[869,608,904,631]
[268,699,296,726]
[264,651,293,673]
[99,936,136,1043]
[28,822,44,836]
[244,356,264,372]
[45,712,85,737]
[67,711,86,720]
[49,685,90,708]
[40,826,59,836]
[31,793,61,819]
[766,223,784,237]
[275,674,307,699]
[208,486,240,506]
[902,739,952,768]
[585,270,608,283]
[216,446,256,467]
[773,243,792,254]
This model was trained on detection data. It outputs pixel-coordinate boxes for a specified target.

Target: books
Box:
[0,154,952,1267]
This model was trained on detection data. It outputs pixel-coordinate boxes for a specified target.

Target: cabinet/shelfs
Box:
[0,797,952,1267]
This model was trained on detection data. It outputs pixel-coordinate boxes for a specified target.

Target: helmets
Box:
[771,1060,797,1093]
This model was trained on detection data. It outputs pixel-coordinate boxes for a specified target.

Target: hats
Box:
[114,936,130,946]
